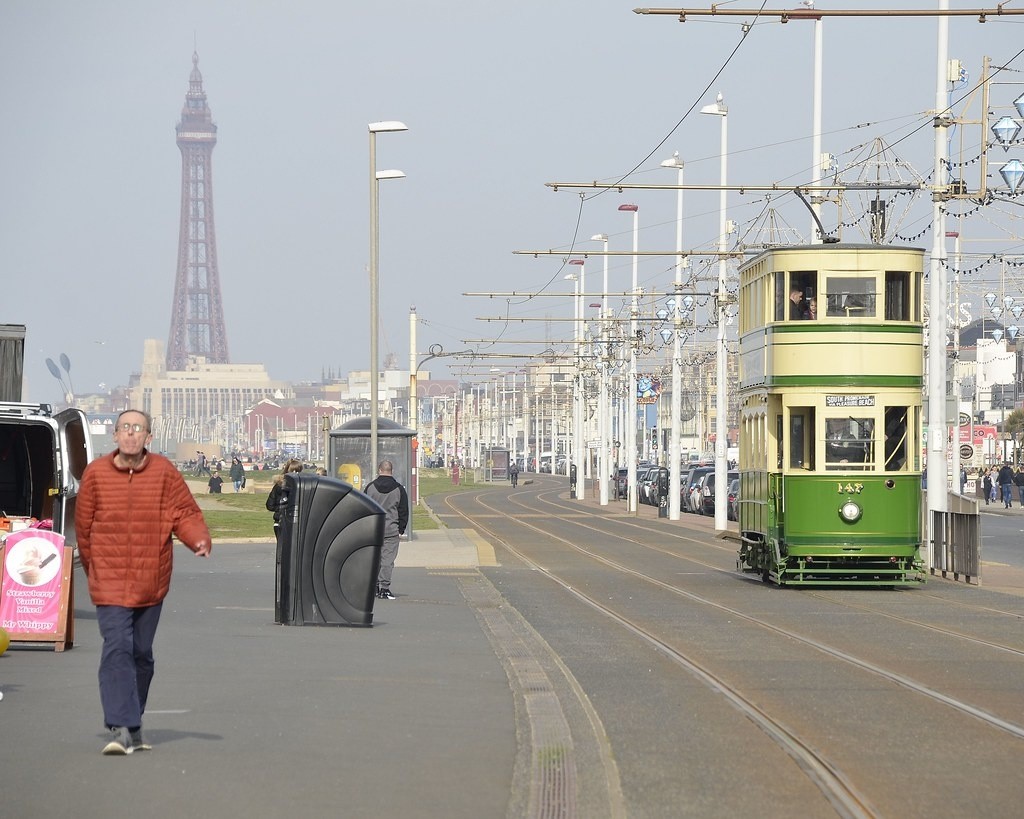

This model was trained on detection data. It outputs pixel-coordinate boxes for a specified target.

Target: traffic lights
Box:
[651,428,658,450]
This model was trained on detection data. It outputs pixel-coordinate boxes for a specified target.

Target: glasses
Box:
[114,423,150,433]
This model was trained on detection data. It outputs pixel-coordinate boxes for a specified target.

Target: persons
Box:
[310,463,317,469]
[208,471,223,493]
[267,459,303,538]
[74,409,212,755]
[960,464,968,494]
[510,463,519,485]
[981,462,1024,510]
[315,466,327,476]
[922,465,927,489]
[217,459,225,472]
[827,419,858,470]
[248,456,279,470]
[229,457,245,492]
[363,460,409,599]
[183,451,211,478]
[430,452,443,469]
[776,281,817,319]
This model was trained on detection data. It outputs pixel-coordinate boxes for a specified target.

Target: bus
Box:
[736,244,928,589]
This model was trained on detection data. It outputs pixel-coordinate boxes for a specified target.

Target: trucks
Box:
[0,402,95,570]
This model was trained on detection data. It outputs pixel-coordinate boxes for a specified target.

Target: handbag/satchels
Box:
[241,476,246,489]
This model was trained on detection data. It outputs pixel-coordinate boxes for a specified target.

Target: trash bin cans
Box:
[273,472,388,627]
[608,480,615,501]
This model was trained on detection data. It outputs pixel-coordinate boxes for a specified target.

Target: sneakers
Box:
[101,723,144,753]
[376,588,395,599]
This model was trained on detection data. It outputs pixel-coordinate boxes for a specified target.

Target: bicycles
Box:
[512,473,517,488]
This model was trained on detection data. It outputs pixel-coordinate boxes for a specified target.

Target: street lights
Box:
[560,357,570,475]
[565,273,578,464]
[590,233,610,505]
[247,414,265,460]
[619,203,637,512]
[700,103,728,530]
[371,120,409,484]
[590,303,602,480]
[418,361,556,474]
[662,157,685,522]
[570,260,585,500]
[787,8,823,245]
[315,411,319,460]
[945,231,962,497]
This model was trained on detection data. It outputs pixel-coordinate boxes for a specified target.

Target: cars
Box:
[613,461,739,522]
[517,451,572,475]
